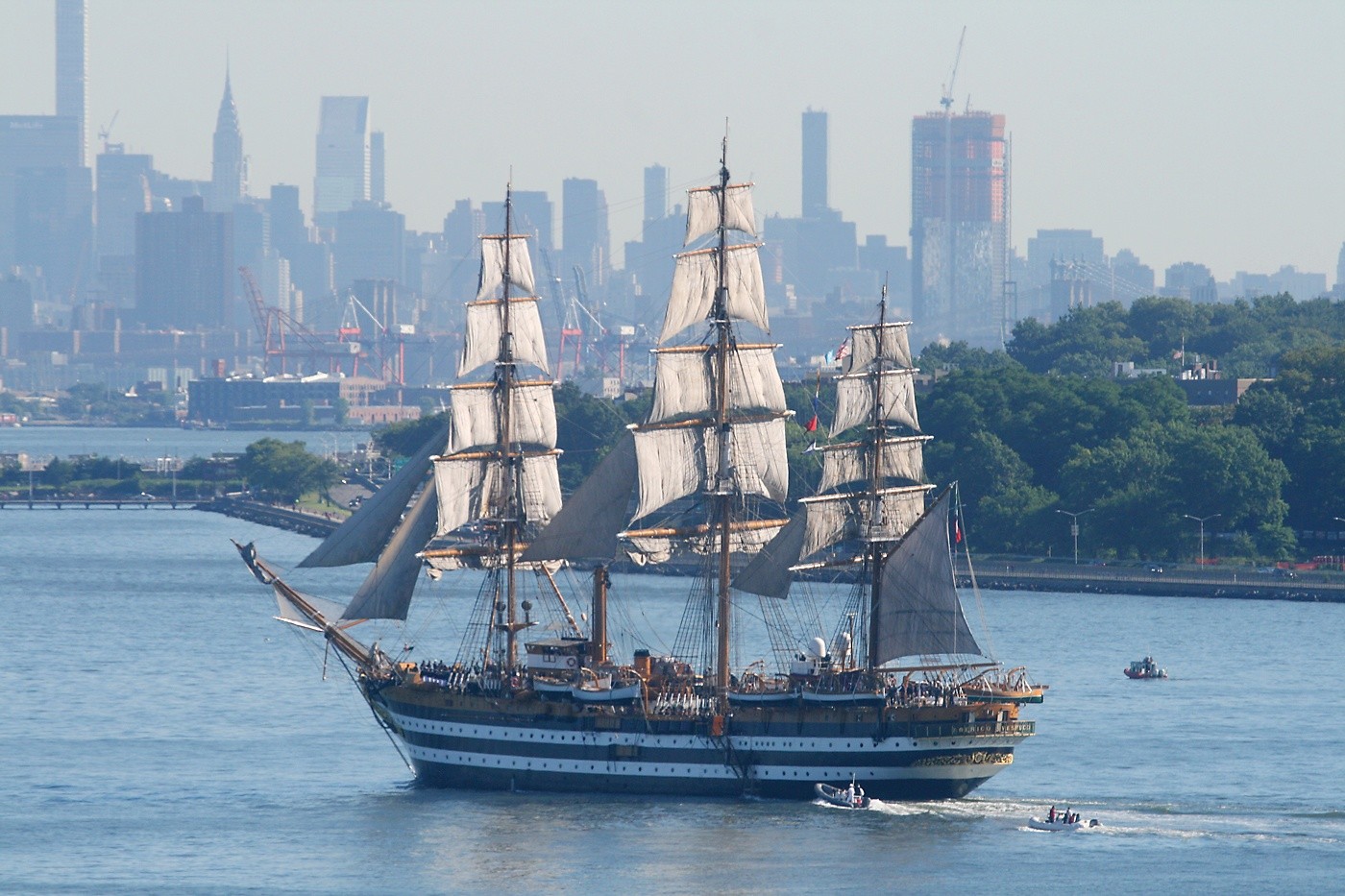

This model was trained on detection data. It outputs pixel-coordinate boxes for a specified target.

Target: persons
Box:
[831,783,865,804]
[1063,807,1080,824]
[888,673,942,704]
[1050,805,1055,823]
[404,658,469,692]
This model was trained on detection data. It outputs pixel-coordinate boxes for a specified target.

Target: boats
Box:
[532,672,612,704]
[726,684,800,708]
[569,678,642,709]
[813,781,871,809]
[800,684,888,711]
[1029,815,1102,833]
[961,678,1046,706]
[1123,661,1169,679]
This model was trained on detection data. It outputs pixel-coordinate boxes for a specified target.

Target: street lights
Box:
[1055,508,1096,564]
[1183,513,1222,571]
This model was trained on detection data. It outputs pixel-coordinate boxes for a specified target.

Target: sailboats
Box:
[226,109,1054,805]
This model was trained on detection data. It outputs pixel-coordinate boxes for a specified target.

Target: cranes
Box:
[236,264,411,406]
[537,242,637,400]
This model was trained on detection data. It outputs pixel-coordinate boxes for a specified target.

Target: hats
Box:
[849,784,854,787]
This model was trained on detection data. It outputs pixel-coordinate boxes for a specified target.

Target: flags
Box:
[814,386,820,412]
[952,502,963,545]
[825,351,835,364]
[835,340,850,359]
[803,415,818,431]
